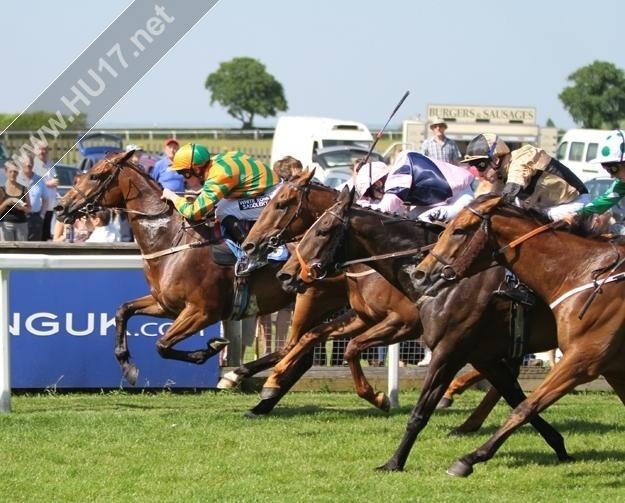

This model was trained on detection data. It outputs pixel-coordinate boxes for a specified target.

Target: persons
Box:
[430,133,589,223]
[560,130,625,239]
[0,116,483,243]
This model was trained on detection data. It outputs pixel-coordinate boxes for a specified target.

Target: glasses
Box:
[472,159,493,172]
[183,170,193,179]
[602,165,621,175]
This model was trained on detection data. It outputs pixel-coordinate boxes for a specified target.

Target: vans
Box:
[553,127,625,182]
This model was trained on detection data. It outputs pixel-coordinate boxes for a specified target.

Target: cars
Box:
[0,132,163,208]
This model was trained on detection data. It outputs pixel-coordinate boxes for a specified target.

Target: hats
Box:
[427,116,449,130]
[163,139,179,146]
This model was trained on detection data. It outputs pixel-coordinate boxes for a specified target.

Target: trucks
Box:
[401,104,542,168]
[271,114,416,181]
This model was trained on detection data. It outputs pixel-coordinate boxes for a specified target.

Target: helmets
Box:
[461,132,510,164]
[595,130,625,164]
[355,160,389,200]
[166,143,210,172]
[126,143,144,152]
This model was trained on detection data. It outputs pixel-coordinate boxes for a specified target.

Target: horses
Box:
[52,149,347,388]
[275,185,571,472]
[241,167,485,413]
[413,193,625,478]
